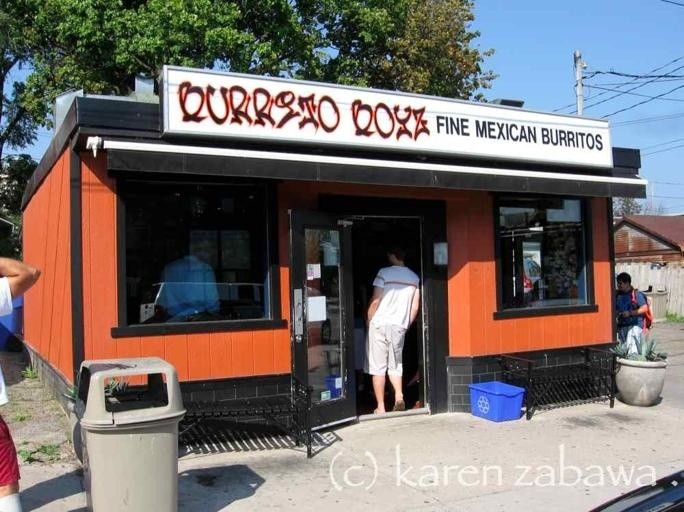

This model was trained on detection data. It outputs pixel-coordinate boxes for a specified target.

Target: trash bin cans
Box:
[641,291,668,323]
[74,357,186,512]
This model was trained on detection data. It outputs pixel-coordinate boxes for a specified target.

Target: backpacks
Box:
[632,288,653,332]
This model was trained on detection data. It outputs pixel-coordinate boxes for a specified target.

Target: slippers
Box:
[392,399,405,411]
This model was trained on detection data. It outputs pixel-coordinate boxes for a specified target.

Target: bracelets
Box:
[629,310,633,317]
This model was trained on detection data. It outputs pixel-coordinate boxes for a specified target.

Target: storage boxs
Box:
[469,380,526,422]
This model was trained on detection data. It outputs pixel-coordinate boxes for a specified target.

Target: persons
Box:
[156,238,220,322]
[0,256,42,512]
[363,245,421,414]
[616,273,652,354]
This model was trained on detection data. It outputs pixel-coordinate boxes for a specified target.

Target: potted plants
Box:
[608,333,670,407]
